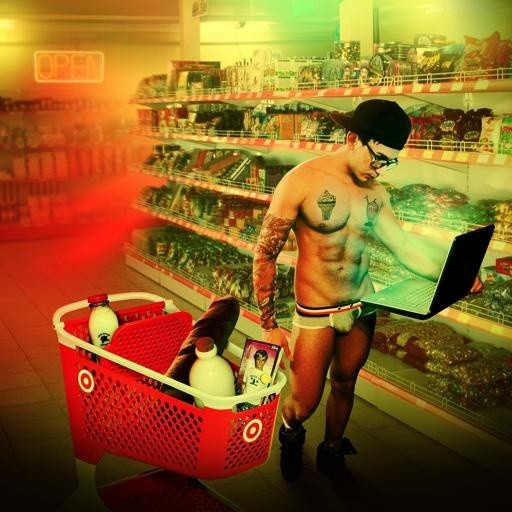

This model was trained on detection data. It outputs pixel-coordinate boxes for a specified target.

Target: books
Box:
[236,338,285,411]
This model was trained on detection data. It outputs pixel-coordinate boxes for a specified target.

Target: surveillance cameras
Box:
[239,21,246,27]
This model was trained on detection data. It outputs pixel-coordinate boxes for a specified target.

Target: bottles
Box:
[189,336,237,434]
[388,54,412,84]
[87,293,120,364]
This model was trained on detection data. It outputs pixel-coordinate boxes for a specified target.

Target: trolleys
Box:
[52,291,287,512]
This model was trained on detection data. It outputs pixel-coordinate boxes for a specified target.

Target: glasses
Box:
[365,143,398,171]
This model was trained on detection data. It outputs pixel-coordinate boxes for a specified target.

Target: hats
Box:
[329,99,411,150]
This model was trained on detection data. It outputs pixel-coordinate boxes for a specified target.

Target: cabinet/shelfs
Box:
[0,68,512,476]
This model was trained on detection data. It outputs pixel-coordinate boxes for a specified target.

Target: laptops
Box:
[360,223,495,317]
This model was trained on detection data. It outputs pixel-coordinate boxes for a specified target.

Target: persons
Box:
[252,100,484,494]
[241,349,268,409]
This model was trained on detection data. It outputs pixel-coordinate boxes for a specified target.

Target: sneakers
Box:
[278,423,307,482]
[316,437,360,502]
